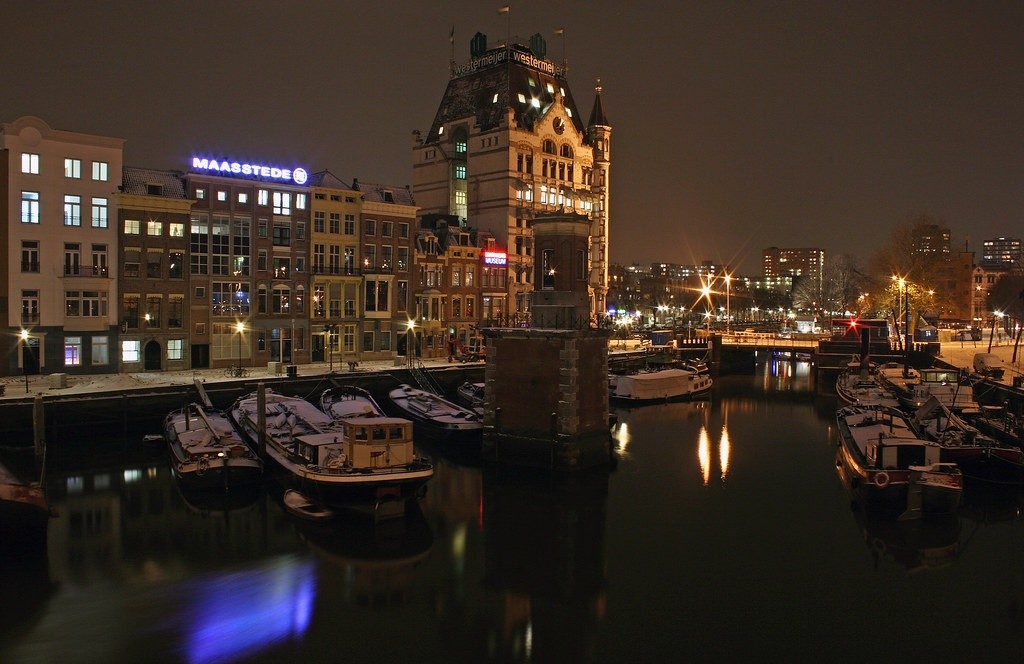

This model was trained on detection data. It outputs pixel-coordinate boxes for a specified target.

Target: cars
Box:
[948,325,967,330]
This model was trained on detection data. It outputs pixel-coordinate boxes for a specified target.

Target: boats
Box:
[829,358,1024,520]
[388,382,484,447]
[812,318,891,375]
[165,403,262,514]
[608,360,714,406]
[457,379,619,431]
[228,385,435,525]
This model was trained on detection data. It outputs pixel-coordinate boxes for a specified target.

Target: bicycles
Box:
[224,365,250,378]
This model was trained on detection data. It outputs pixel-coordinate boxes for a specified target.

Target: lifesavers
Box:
[874,472,890,487]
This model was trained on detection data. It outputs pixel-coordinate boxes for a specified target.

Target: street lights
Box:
[899,278,904,335]
[236,322,243,377]
[408,319,414,366]
[21,329,29,393]
[724,275,730,335]
